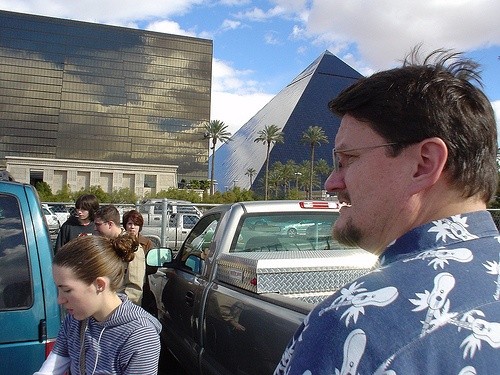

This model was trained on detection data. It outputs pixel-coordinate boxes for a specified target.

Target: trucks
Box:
[135,197,246,253]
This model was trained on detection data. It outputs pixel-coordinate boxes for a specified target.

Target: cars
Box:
[283,220,323,237]
[42,202,70,228]
[306,224,334,241]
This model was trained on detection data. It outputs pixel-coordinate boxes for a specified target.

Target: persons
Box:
[93,205,145,308]
[123,210,153,256]
[272,41,500,375]
[34,232,162,375]
[54,195,100,255]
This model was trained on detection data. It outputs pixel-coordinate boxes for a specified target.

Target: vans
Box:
[0,181,64,375]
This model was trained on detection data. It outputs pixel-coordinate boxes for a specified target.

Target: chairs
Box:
[307,224,335,250]
[245,236,286,251]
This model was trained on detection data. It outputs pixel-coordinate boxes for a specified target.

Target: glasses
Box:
[331,143,395,173]
[95,220,110,227]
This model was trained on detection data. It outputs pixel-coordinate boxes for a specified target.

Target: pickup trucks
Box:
[142,200,381,375]
